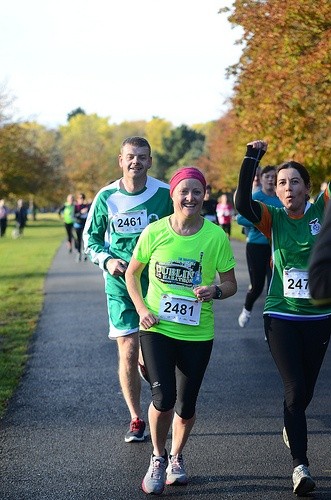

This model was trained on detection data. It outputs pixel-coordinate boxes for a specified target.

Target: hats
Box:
[169,167,206,198]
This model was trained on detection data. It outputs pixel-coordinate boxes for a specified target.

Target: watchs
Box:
[213,285,222,299]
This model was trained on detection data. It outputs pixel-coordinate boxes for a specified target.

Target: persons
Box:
[200,186,232,238]
[234,166,263,291]
[125,166,237,496]
[58,193,92,260]
[236,165,285,341]
[236,140,331,493]
[0,198,8,237]
[83,138,174,442]
[14,199,28,236]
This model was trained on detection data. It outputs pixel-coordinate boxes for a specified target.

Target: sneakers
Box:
[142,448,168,495]
[283,427,290,448]
[238,306,251,328]
[124,417,148,442]
[292,465,315,496]
[165,454,188,485]
[138,363,150,383]
